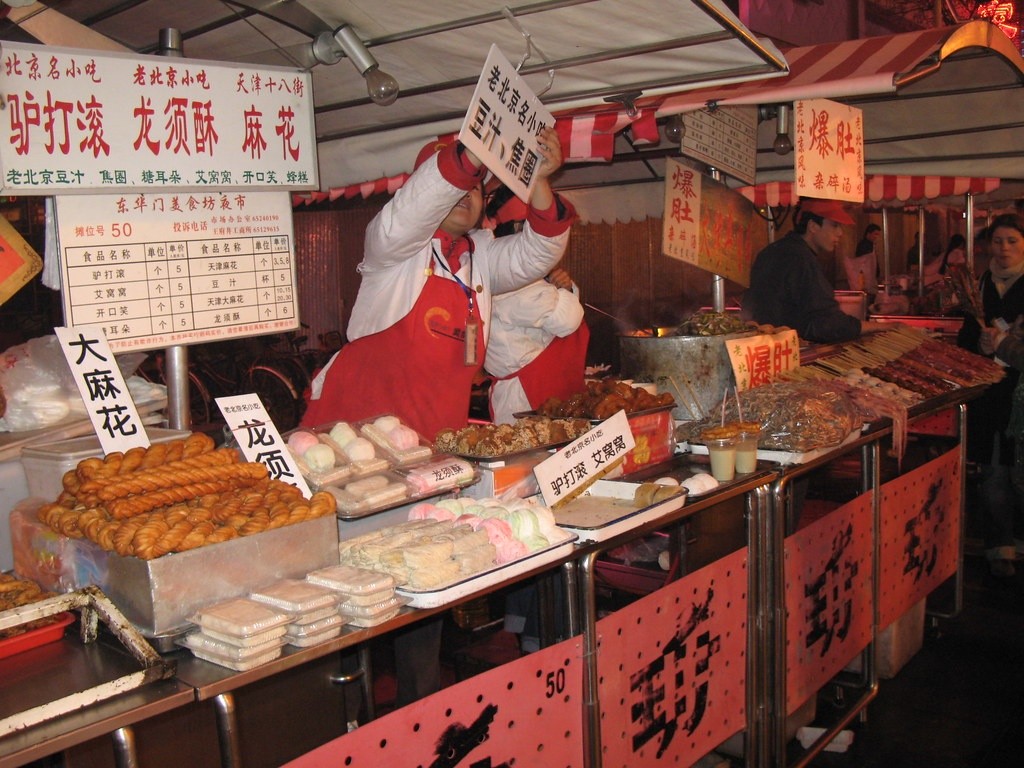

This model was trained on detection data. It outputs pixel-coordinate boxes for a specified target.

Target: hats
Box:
[414,141,451,171]
[796,198,855,225]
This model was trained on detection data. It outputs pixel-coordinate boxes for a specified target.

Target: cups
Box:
[709,439,737,481]
[729,437,759,474]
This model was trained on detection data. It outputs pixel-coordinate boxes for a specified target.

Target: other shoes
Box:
[991,559,1016,578]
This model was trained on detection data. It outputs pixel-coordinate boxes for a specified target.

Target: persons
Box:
[749,197,903,343]
[939,233,966,274]
[855,223,882,275]
[960,211,1024,581]
[299,125,592,716]
[907,232,919,264]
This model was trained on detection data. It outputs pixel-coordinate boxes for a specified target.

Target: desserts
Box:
[289,415,564,592]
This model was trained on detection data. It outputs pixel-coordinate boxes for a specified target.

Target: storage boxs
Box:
[716,692,816,763]
[839,592,926,681]
[10,493,341,656]
